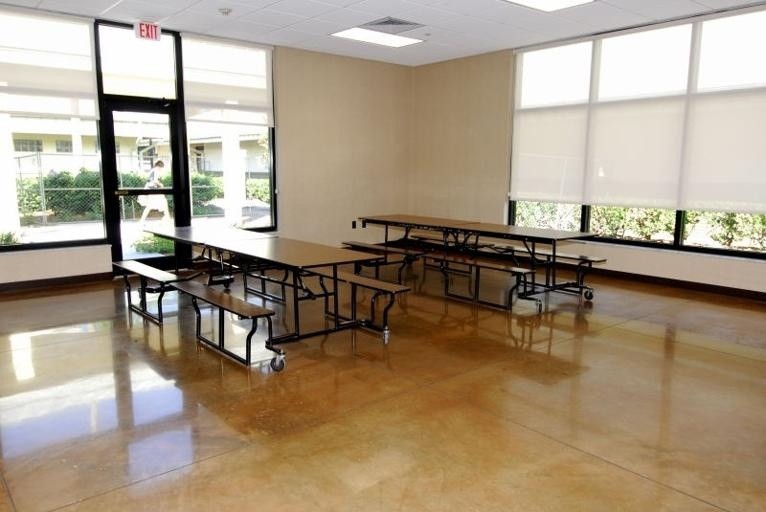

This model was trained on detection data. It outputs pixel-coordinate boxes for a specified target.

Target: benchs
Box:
[341,232,607,312]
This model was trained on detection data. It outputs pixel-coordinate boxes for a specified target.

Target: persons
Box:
[135,160,172,231]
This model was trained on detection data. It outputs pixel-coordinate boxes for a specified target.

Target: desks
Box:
[358,214,600,312]
[135,224,384,355]
